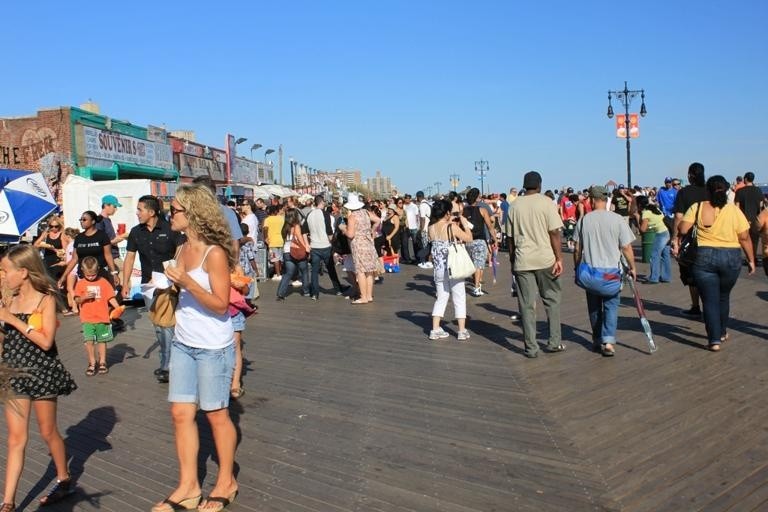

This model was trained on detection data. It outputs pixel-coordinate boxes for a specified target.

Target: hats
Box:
[664,176,672,183]
[342,193,366,212]
[102,195,122,208]
[567,187,573,192]
[618,184,625,189]
[588,185,609,200]
[522,172,542,191]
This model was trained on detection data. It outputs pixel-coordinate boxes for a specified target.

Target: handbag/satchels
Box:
[676,223,699,269]
[288,235,308,260]
[446,242,478,281]
[147,283,182,328]
[574,256,622,298]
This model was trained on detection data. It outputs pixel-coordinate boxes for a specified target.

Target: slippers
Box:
[195,490,237,512]
[367,297,374,303]
[148,493,202,512]
[350,298,369,305]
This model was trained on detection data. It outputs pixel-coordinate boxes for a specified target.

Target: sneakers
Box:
[428,326,450,340]
[679,305,702,318]
[456,327,471,341]
[417,261,436,270]
[472,286,485,297]
[659,279,671,283]
[154,368,170,384]
[525,350,539,359]
[642,279,659,285]
[547,342,568,353]
[270,273,283,282]
[291,279,302,286]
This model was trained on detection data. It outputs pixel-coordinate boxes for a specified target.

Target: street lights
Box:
[450,173,460,192]
[289,154,328,197]
[434,182,442,193]
[419,186,432,196]
[607,76,648,202]
[474,158,489,195]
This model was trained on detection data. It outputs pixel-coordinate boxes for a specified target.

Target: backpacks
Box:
[300,216,310,234]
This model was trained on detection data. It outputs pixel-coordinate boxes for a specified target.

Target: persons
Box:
[217,195,359,300]
[671,165,709,318]
[151,182,239,512]
[572,186,637,358]
[36,193,122,375]
[428,200,473,340]
[607,184,657,236]
[219,238,254,402]
[500,171,567,358]
[192,175,244,264]
[676,174,755,351]
[0,242,76,512]
[632,196,671,283]
[448,188,525,296]
[546,187,593,250]
[339,194,381,303]
[364,191,439,283]
[649,178,682,236]
[121,195,184,383]
[726,172,768,275]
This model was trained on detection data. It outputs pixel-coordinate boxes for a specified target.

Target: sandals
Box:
[703,342,722,352]
[591,342,606,351]
[38,472,78,506]
[719,331,731,342]
[0,500,17,512]
[602,343,617,358]
[98,361,109,375]
[86,360,98,377]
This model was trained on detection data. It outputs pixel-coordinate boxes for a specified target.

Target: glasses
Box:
[169,205,186,216]
[672,183,681,186]
[79,218,91,222]
[47,224,60,229]
[241,203,251,206]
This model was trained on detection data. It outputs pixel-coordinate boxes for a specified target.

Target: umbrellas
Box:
[0,168,60,242]
